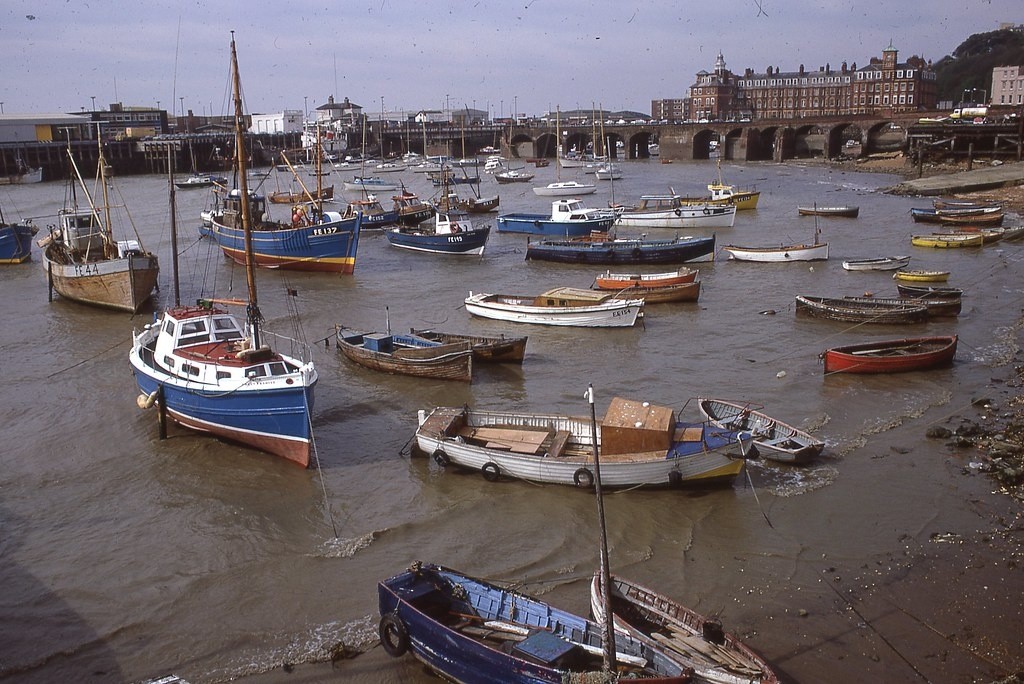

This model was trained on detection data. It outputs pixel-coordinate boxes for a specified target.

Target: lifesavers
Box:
[632,248,641,256]
[433,449,450,467]
[573,468,594,489]
[606,249,614,258]
[380,614,409,658]
[482,462,500,482]
[703,208,709,215]
[577,252,585,261]
[449,222,459,233]
[728,199,733,203]
[675,209,681,215]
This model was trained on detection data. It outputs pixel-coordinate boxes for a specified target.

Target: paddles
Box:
[852,345,918,355]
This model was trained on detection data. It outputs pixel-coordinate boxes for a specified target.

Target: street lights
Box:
[961,87,988,106]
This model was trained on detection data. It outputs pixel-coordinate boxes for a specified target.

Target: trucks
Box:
[950,107,990,119]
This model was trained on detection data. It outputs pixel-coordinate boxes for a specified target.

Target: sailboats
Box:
[174,90,624,236]
[126,26,320,469]
[37,118,160,315]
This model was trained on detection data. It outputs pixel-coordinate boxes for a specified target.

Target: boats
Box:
[209,144,365,276]
[893,268,951,282]
[589,266,701,302]
[0,175,40,266]
[697,395,827,466]
[798,205,860,219]
[719,200,830,263]
[455,286,646,331]
[376,382,696,684]
[906,197,1005,249]
[794,283,963,325]
[314,323,473,384]
[680,157,761,210]
[842,255,912,271]
[522,229,717,267]
[379,165,492,257]
[590,568,780,684]
[818,333,959,375]
[607,193,737,228]
[404,326,530,366]
[397,399,754,491]
